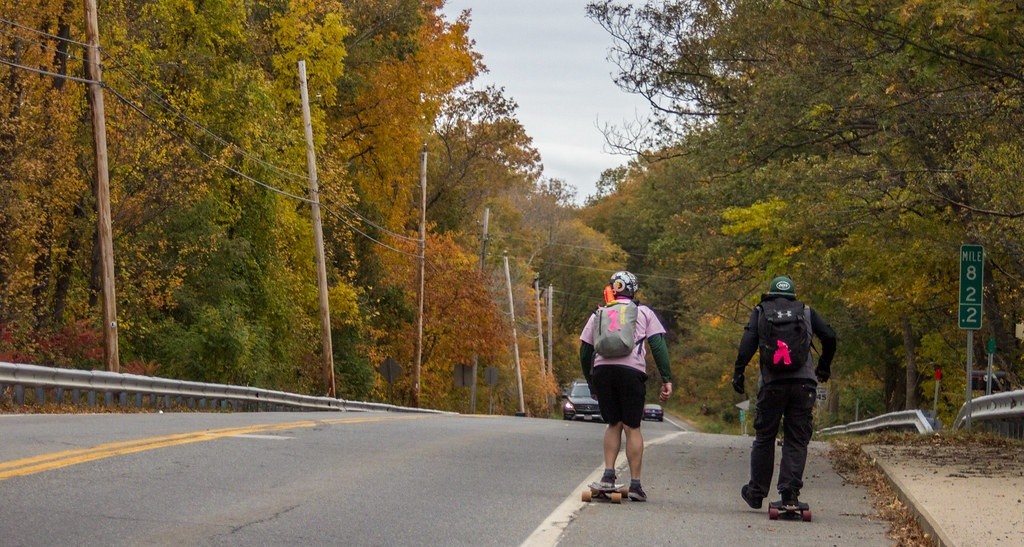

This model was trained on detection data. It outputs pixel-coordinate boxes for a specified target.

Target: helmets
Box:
[768,276,797,297]
[610,270,638,296]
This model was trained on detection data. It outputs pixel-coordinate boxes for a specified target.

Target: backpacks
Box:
[758,301,812,374]
[592,298,647,355]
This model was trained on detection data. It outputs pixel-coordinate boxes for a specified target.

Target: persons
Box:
[732,275,837,510]
[579,270,673,501]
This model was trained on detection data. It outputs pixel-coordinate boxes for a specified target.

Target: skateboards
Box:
[768,500,811,522]
[582,481,629,504]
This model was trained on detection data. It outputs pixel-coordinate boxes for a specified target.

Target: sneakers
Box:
[600,476,616,488]
[782,493,797,505]
[741,485,762,508]
[628,486,647,501]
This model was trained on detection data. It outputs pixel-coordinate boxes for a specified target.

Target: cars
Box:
[643,404,663,421]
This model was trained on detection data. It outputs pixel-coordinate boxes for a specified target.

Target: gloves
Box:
[815,365,831,382]
[732,372,745,394]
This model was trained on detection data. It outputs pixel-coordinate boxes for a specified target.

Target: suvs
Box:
[562,381,603,421]
